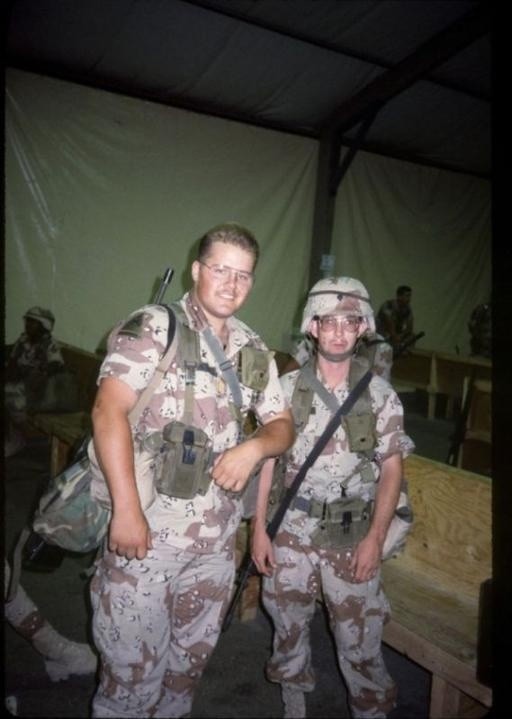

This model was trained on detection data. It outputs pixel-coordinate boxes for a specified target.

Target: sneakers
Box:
[45,642,99,682]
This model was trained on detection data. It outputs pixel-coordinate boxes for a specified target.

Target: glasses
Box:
[320,316,363,333]
[198,260,256,292]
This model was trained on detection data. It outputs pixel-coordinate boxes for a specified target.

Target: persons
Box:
[2,555,100,718]
[278,322,397,386]
[465,299,492,380]
[374,284,419,358]
[246,272,421,719]
[81,220,295,719]
[1,304,70,459]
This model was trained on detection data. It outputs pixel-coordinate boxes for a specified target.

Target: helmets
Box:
[23,306,56,333]
[300,277,375,338]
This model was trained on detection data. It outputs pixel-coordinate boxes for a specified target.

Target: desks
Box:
[38,407,491,717]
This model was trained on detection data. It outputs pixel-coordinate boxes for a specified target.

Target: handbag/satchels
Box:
[31,454,113,554]
[378,477,413,562]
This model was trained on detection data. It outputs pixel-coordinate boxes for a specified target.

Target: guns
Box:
[220,551,256,635]
[21,271,177,568]
